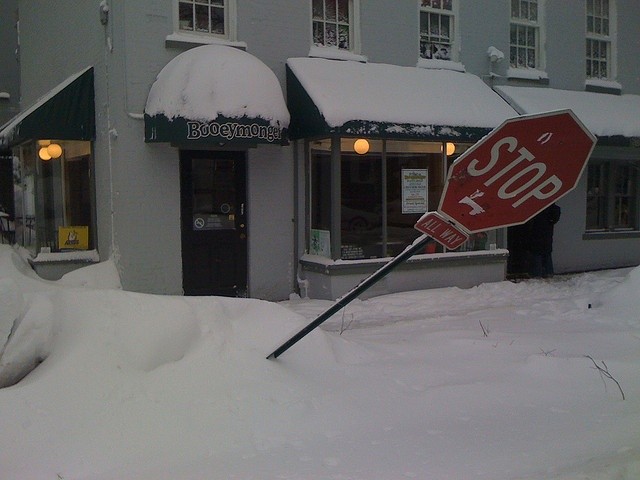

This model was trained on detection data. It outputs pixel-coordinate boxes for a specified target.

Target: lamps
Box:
[442,142,456,156]
[47,143,62,158]
[353,138,370,155]
[39,146,50,160]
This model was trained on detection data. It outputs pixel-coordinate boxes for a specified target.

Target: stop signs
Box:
[437,109,598,234]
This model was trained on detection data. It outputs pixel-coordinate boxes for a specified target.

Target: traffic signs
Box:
[414,211,468,250]
